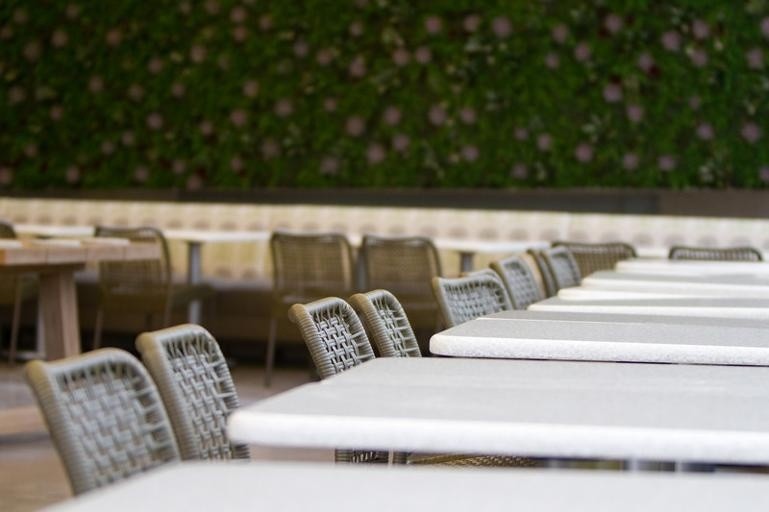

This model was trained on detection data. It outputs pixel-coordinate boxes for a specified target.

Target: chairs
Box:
[551,241,638,284]
[525,246,582,293]
[348,286,534,467]
[432,274,516,332]
[23,346,184,500]
[361,235,447,338]
[289,294,391,463]
[0,223,41,356]
[136,324,253,461]
[90,224,217,354]
[264,231,356,390]
[488,258,545,310]
[670,245,763,263]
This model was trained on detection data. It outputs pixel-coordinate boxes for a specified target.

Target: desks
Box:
[642,245,669,259]
[349,233,548,280]
[427,308,769,367]
[527,258,769,318]
[13,219,274,322]
[41,458,766,512]
[224,356,769,481]
